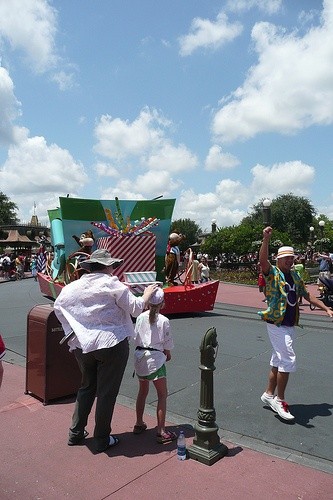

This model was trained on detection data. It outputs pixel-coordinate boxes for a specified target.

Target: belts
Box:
[321,270,328,272]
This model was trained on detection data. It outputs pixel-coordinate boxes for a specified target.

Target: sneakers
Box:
[269,397,296,420]
[155,429,177,443]
[134,422,146,433]
[261,392,274,405]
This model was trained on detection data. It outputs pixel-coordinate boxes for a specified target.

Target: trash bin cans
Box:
[26,304,76,406]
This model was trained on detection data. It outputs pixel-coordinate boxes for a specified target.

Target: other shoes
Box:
[316,295,323,298]
[318,296,326,300]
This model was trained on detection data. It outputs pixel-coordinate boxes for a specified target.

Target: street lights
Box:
[211,219,217,233]
[259,197,273,228]
[309,226,315,253]
[318,219,326,254]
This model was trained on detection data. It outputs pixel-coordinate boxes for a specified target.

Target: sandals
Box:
[77,438,89,444]
[93,436,120,454]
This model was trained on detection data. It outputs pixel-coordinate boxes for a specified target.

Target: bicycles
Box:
[0,264,26,283]
[309,285,333,311]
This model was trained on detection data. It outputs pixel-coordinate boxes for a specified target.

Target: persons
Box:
[259,227,333,420]
[0,333,7,386]
[131,287,177,442]
[0,232,333,317]
[53,250,159,451]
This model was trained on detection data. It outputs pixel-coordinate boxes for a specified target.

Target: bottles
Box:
[177,431,186,461]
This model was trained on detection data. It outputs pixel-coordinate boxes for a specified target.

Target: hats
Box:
[274,246,299,260]
[79,249,123,272]
[148,288,165,304]
